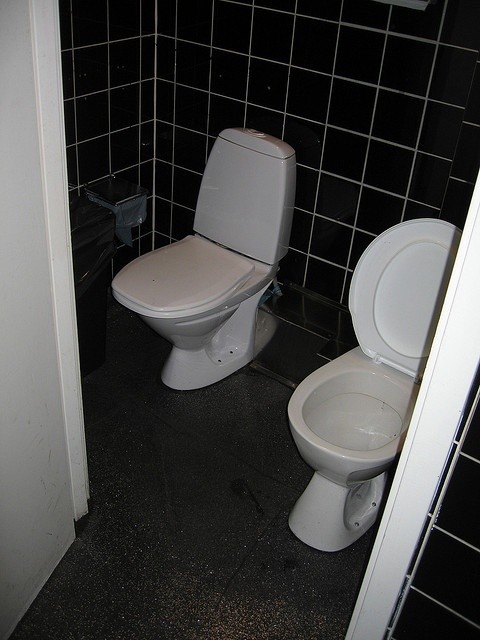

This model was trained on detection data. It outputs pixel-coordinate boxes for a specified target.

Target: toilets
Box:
[111,127,297,390]
[286,218,462,553]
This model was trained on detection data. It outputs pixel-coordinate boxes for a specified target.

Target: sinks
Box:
[70,195,116,299]
